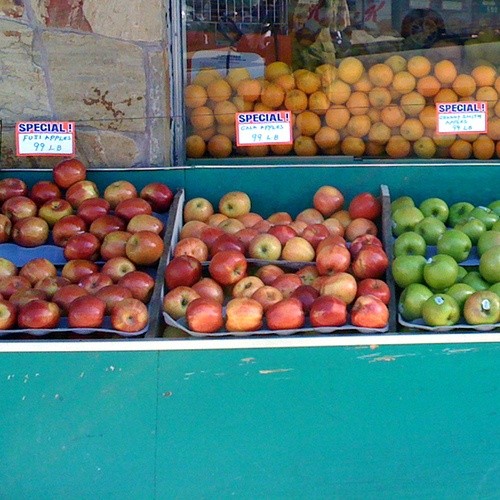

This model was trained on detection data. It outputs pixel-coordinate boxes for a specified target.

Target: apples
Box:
[0,158,173,332]
[164,185,391,333]
[390,196,500,328]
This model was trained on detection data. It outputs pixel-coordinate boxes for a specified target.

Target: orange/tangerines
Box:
[184,56,500,160]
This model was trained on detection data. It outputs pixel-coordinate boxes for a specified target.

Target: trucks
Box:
[331,0,473,48]
